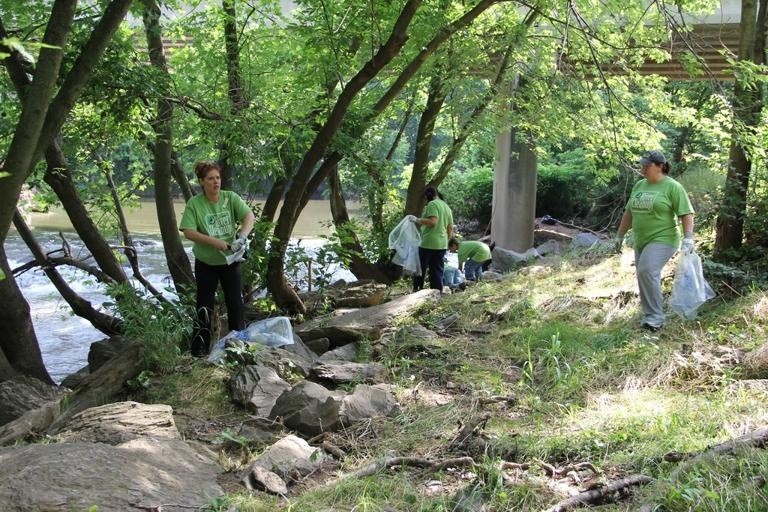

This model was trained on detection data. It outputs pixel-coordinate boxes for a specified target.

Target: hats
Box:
[639,150,666,165]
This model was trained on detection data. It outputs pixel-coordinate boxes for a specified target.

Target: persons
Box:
[616,151,694,329]
[179,161,255,356]
[409,187,454,291]
[449,237,492,282]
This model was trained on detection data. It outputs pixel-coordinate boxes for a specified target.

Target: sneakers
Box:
[645,323,664,333]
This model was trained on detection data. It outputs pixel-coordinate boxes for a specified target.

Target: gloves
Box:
[614,235,624,254]
[231,238,244,252]
[681,238,694,254]
